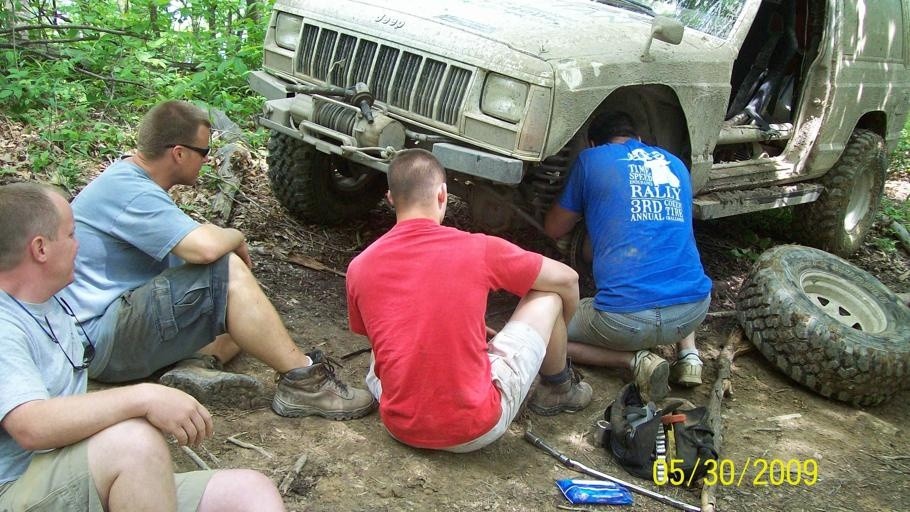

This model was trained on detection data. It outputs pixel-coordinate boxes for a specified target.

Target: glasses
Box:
[165,143,211,158]
[45,298,96,371]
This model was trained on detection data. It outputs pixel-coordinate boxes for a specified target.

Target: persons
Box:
[349,146,596,456]
[1,182,289,510]
[60,100,384,418]
[542,110,712,403]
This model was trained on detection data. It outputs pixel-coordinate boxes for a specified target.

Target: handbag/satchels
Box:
[604,384,719,482]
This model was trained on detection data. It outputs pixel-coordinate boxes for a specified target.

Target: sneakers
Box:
[159,353,269,409]
[632,352,669,405]
[272,353,376,421]
[530,358,594,414]
[669,361,702,384]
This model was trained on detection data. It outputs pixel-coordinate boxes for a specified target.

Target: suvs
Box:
[242,0,905,257]
